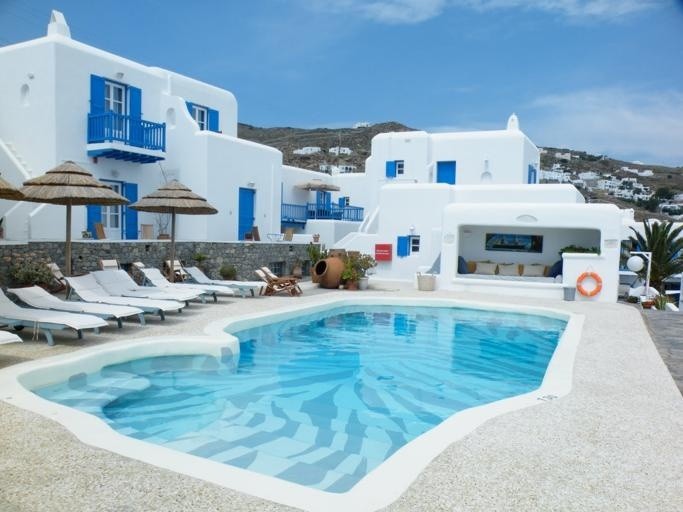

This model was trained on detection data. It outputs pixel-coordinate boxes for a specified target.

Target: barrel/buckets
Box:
[417,271,437,291]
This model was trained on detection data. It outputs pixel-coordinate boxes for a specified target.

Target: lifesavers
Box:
[577,272,603,297]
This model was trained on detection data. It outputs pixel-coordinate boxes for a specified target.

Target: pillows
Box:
[458,256,562,277]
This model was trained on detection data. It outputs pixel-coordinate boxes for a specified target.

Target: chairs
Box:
[139,266,233,303]
[182,264,267,298]
[65,268,206,322]
[98,258,190,281]
[42,259,71,294]
[0,289,109,345]
[6,284,146,335]
[253,265,302,298]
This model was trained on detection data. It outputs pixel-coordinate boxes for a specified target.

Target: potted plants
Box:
[307,242,378,290]
